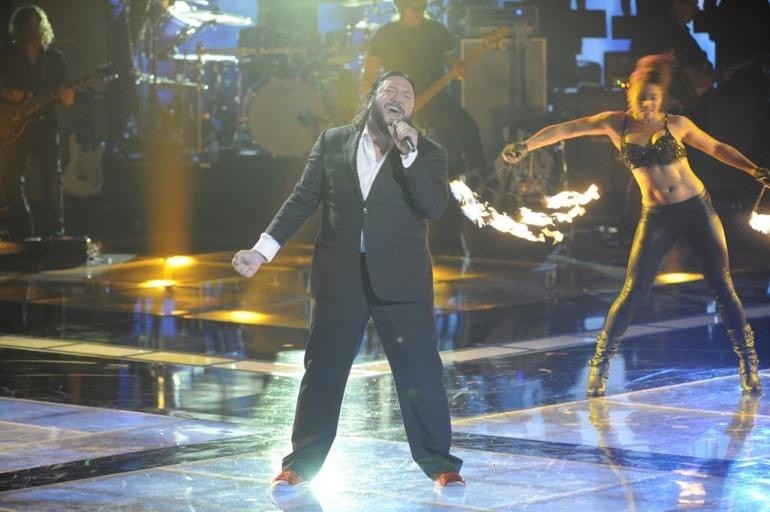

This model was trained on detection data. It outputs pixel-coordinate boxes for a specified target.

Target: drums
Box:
[241,75,332,158]
[323,30,354,65]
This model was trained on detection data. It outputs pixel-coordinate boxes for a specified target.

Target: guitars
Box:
[489,23,562,197]
[63,105,106,197]
[663,52,763,109]
[1,61,122,145]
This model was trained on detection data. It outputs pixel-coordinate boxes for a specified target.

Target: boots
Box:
[726,322,762,393]
[587,328,625,397]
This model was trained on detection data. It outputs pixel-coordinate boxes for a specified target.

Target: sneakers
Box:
[271,468,307,492]
[435,469,465,490]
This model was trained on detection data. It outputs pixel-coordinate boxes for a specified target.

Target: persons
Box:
[231,70,469,494]
[498,54,768,401]
[1,4,76,243]
[362,0,472,141]
[587,399,759,512]
[614,0,734,251]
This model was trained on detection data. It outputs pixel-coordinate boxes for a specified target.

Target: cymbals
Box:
[182,10,253,27]
[172,54,236,65]
